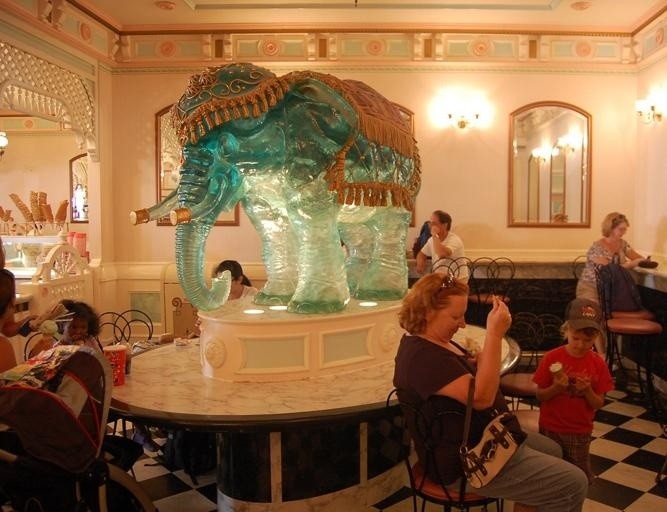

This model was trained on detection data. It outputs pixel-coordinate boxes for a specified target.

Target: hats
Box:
[569,299,603,333]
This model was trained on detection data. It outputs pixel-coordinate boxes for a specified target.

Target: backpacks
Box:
[598,252,644,312]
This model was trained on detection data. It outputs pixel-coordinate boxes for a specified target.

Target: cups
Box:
[103,344,127,387]
[62,231,87,274]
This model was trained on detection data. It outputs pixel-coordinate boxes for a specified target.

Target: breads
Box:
[461,337,482,354]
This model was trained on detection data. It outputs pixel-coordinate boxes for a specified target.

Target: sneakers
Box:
[131,428,160,457]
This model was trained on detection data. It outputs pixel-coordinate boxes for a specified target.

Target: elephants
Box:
[130,62,423,314]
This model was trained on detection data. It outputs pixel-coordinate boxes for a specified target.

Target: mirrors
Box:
[508,102,593,228]
[69,153,89,223]
[154,102,240,226]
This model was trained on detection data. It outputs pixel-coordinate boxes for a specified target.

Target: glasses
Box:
[435,273,454,296]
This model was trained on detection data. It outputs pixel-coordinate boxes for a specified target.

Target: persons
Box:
[0,238,39,337]
[191,260,258,335]
[414,211,467,290]
[395,270,589,510]
[48,299,161,454]
[530,295,613,473]
[573,212,645,377]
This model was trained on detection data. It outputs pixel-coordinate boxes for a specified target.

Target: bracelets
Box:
[432,233,440,239]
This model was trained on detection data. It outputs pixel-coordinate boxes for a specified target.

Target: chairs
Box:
[23,309,155,484]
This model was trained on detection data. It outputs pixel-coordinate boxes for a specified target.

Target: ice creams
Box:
[39,319,59,339]
[549,361,565,380]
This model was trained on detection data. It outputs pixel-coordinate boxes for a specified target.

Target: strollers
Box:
[0,345,154,512]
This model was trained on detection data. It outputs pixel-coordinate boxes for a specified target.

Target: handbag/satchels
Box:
[0,345,112,472]
[158,428,216,475]
[460,412,528,487]
[414,221,433,259]
